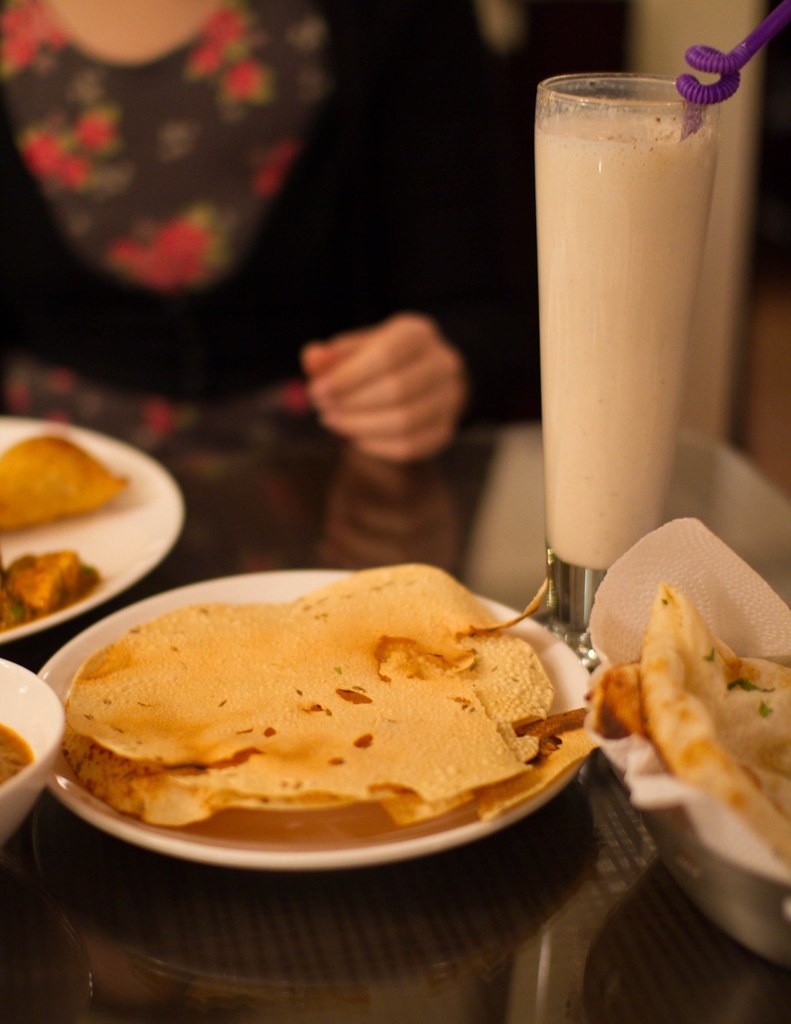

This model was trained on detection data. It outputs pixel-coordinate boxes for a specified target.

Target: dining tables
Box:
[0,419,791,1024]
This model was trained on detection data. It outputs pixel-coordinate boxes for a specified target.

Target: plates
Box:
[0,416,184,643]
[39,571,585,869]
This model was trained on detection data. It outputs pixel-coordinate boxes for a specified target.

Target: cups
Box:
[535,74,719,670]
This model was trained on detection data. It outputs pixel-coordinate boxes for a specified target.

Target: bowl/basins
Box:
[643,796,791,971]
[0,657,65,849]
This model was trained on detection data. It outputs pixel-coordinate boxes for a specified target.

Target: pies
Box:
[602,583,791,872]
[61,563,592,828]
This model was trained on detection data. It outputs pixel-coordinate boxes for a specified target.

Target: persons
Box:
[0,0,542,462]
